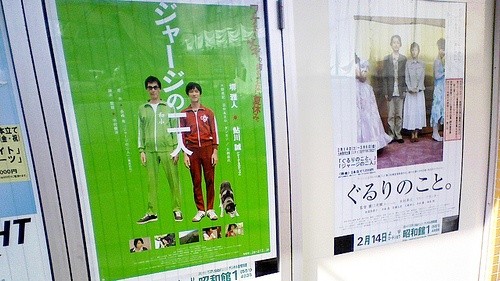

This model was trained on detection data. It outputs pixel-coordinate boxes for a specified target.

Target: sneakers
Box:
[207,210,218,220]
[137,214,158,224]
[192,211,205,221]
[173,211,184,221]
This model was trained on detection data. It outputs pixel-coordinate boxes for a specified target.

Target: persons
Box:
[383,35,407,145]
[203,228,215,241]
[227,224,236,237]
[404,42,427,143]
[130,238,148,253]
[178,82,220,223]
[430,38,445,141]
[136,76,184,225]
[356,54,393,159]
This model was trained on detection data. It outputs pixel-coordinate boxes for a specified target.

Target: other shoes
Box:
[388,139,404,144]
[432,135,442,142]
[411,138,419,142]
